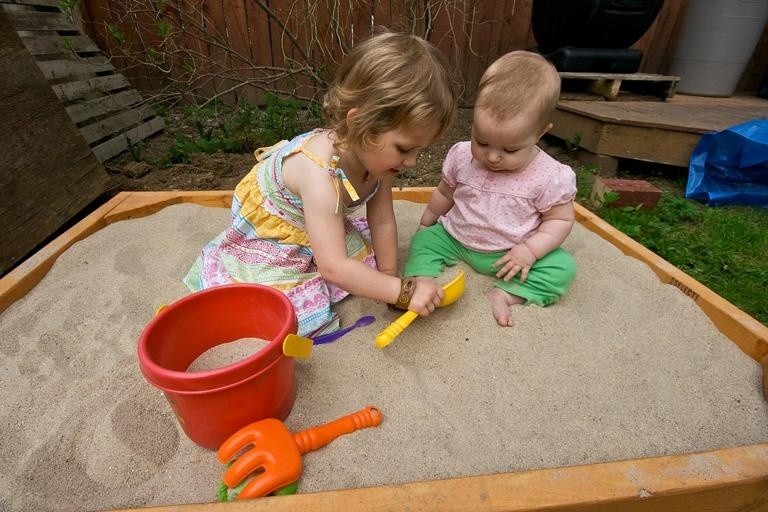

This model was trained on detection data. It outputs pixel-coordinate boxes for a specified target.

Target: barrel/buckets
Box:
[667,0,768,97]
[137,283,316,453]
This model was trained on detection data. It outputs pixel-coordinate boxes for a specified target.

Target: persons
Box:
[172,25,463,338]
[397,46,577,330]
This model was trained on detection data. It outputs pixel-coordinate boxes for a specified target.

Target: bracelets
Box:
[394,275,416,311]
